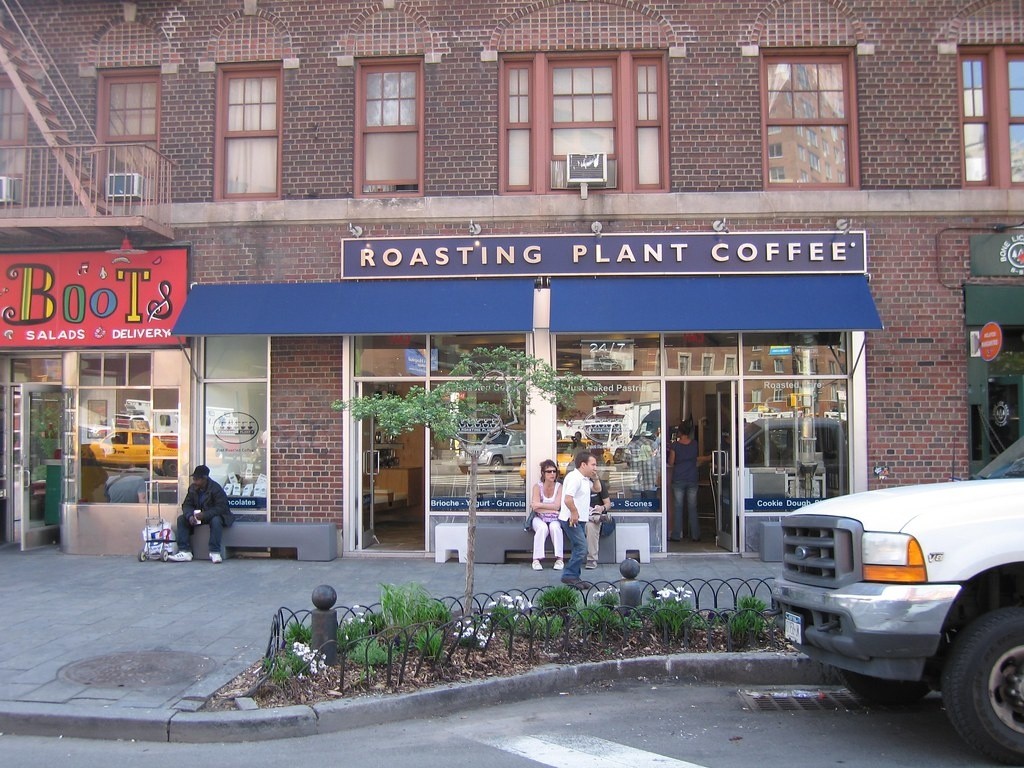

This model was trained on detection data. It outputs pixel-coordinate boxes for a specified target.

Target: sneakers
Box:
[553,560,564,570]
[168,551,193,562]
[601,514,612,522]
[586,560,598,568]
[562,577,590,589]
[532,560,543,570]
[209,552,222,563]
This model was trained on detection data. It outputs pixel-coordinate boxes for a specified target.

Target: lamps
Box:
[712,217,730,233]
[469,220,482,236]
[836,218,853,234]
[591,221,603,239]
[348,223,363,238]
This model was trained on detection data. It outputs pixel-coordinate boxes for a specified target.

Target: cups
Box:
[193,510,202,525]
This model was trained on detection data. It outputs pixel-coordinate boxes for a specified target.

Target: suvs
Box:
[773,432,1024,767]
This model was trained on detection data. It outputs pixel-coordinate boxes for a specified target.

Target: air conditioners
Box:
[108,173,146,200]
[565,154,608,187]
[0,176,15,203]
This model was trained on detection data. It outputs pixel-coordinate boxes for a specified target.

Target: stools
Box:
[615,522,650,564]
[434,523,468,563]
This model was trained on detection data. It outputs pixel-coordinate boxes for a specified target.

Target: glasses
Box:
[543,470,557,473]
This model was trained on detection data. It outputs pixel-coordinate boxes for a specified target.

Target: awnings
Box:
[169,278,538,384]
[548,273,885,377]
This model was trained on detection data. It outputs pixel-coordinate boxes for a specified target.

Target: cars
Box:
[81,428,177,477]
[453,429,527,475]
[520,437,618,484]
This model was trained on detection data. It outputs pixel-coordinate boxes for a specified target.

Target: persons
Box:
[566,431,586,455]
[557,451,602,589]
[624,421,661,513]
[166,465,236,563]
[668,420,701,542]
[583,475,612,569]
[529,460,565,570]
[104,457,147,503]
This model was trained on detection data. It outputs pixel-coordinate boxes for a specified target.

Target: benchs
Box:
[474,524,615,564]
[760,522,783,561]
[171,522,337,561]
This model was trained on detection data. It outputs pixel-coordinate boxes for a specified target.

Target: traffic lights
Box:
[785,394,796,407]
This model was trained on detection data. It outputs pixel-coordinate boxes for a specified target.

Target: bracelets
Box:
[603,506,606,511]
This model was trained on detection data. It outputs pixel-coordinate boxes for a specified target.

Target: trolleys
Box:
[137,481,172,562]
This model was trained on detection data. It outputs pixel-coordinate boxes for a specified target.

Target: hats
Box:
[190,465,210,476]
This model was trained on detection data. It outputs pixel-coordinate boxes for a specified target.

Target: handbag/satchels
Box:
[524,511,536,531]
[601,517,615,536]
[143,518,178,555]
